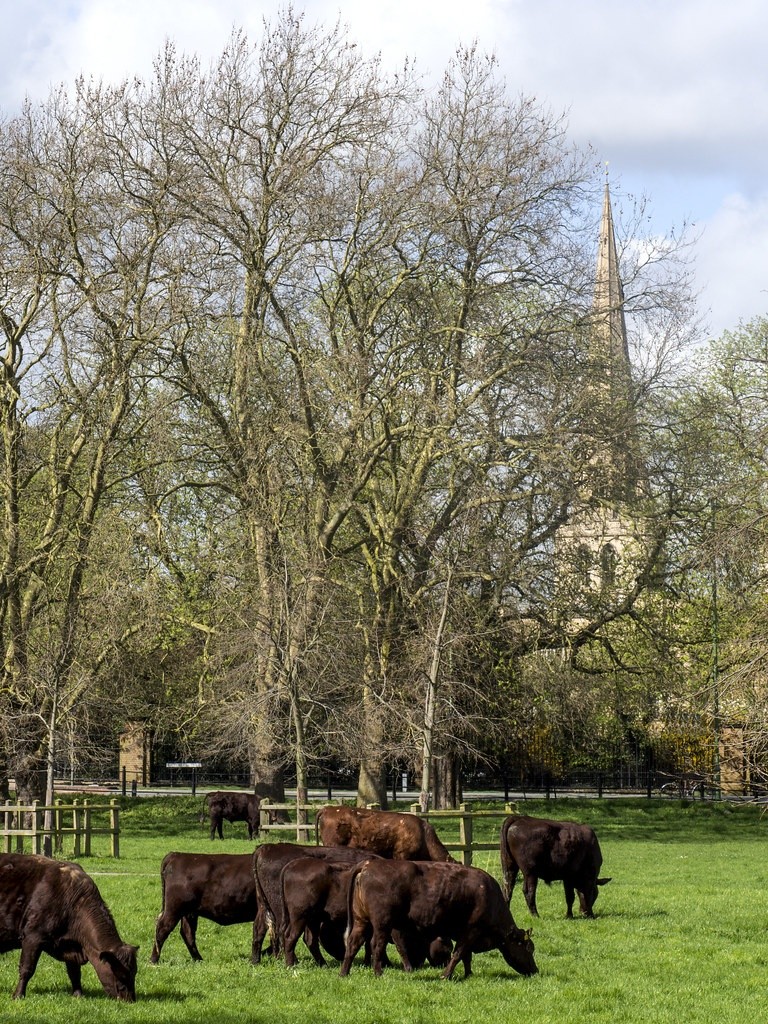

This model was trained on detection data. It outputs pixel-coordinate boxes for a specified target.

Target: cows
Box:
[1,789,616,1005]
